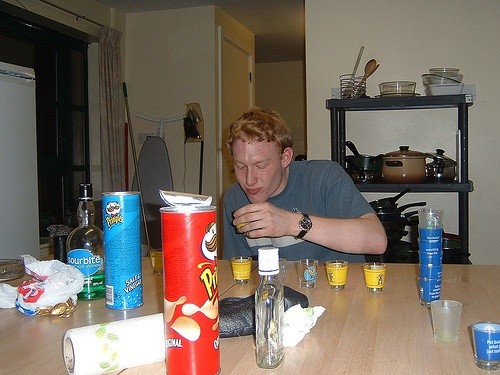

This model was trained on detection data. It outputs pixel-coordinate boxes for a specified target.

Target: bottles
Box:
[66,184,107,302]
[254,247,286,370]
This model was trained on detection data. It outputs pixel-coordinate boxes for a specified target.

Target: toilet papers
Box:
[61,312,165,375]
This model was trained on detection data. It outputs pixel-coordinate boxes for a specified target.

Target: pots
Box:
[344,141,461,264]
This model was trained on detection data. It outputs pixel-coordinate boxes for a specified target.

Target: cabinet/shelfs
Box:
[327,94,474,265]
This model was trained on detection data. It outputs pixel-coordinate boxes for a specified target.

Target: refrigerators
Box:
[0,60,39,265]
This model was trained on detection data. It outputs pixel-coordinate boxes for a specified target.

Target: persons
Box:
[221,106,388,264]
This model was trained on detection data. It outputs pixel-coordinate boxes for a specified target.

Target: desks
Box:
[0,244,500,375]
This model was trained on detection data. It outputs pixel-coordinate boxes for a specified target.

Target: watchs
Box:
[295,214,312,240]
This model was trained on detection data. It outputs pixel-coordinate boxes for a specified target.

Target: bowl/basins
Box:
[377,67,464,96]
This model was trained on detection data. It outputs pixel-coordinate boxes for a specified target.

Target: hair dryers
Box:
[182,110,203,143]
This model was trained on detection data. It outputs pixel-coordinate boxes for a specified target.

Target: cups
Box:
[280,257,286,285]
[234,207,254,234]
[53,234,69,263]
[469,321,499,370]
[325,260,349,291]
[151,248,164,276]
[418,209,443,306]
[296,259,319,289]
[231,255,251,283]
[431,299,463,344]
[363,263,386,293]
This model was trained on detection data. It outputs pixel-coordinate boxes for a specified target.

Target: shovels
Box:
[345,64,379,99]
